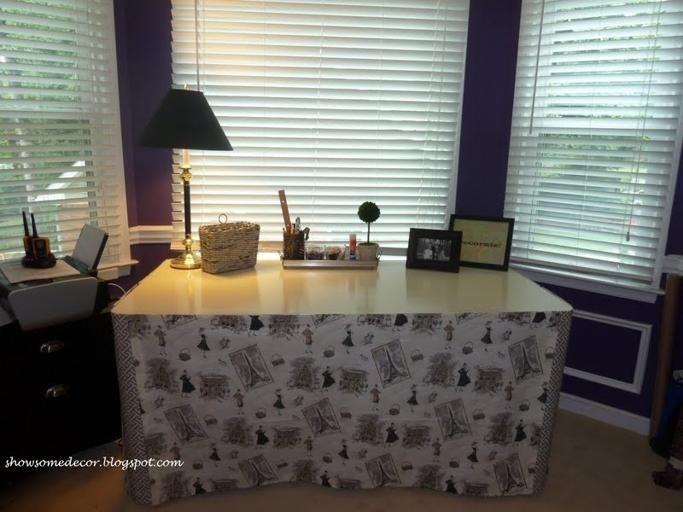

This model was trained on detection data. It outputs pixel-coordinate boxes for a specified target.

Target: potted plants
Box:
[357,201,380,262]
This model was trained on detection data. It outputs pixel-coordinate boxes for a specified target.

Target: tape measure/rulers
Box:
[279,190,291,227]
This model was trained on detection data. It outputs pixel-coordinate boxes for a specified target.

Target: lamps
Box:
[136,89,234,269]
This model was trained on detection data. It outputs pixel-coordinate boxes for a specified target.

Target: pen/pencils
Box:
[283,217,301,234]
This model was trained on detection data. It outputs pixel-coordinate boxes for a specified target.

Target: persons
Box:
[422,238,446,262]
[133,313,551,495]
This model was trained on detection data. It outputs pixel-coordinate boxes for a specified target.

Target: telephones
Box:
[22,211,56,269]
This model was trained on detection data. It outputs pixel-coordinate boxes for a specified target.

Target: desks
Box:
[111,249,573,507]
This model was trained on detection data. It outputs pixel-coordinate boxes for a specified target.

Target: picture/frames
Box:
[449,213,516,271]
[405,228,463,274]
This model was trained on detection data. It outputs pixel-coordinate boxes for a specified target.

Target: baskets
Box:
[199,222,260,274]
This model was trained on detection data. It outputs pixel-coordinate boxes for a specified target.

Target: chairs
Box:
[0,225,109,401]
